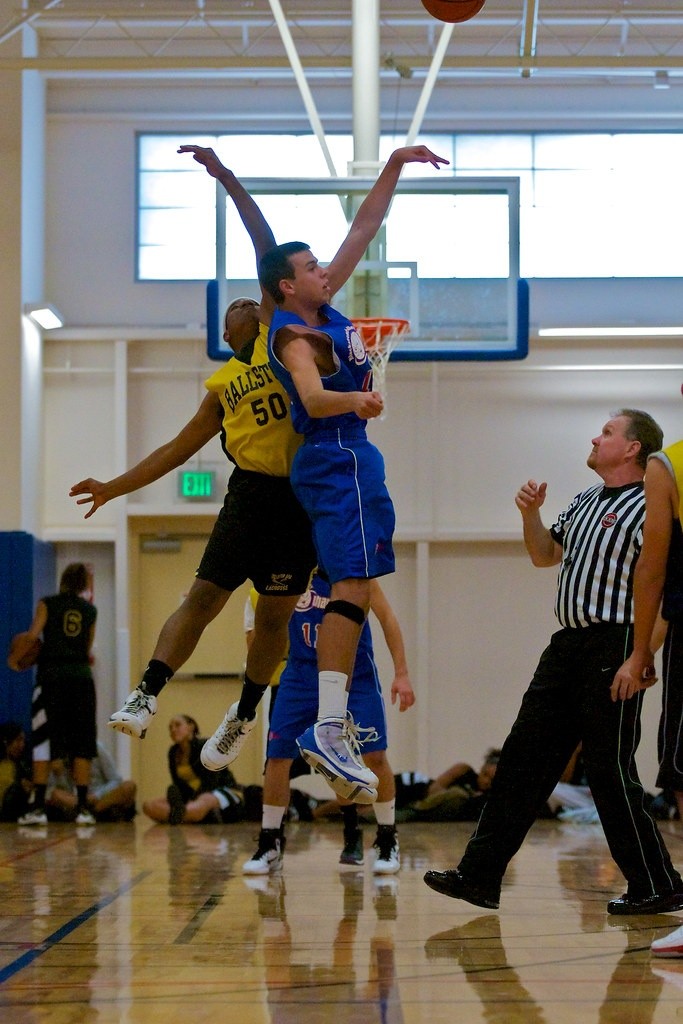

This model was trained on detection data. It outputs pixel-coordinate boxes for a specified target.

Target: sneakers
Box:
[340,828,364,863]
[607,916,683,945]
[200,701,257,770]
[244,876,282,898]
[424,915,502,959]
[338,865,366,886]
[19,812,47,823]
[376,876,397,900]
[77,814,96,824]
[371,830,401,873]
[606,892,683,915]
[18,827,47,838]
[106,687,158,739]
[424,870,501,909]
[324,777,377,803]
[242,836,286,874]
[76,827,96,838]
[295,710,380,788]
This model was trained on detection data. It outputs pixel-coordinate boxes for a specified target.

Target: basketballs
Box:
[421,0,487,24]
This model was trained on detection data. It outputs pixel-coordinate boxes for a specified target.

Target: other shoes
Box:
[651,925,683,957]
[650,959,683,991]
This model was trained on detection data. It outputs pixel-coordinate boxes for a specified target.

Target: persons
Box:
[259,145,450,806]
[7,564,98,825]
[68,144,314,774]
[611,384,683,958]
[0,565,683,875]
[423,410,683,917]
